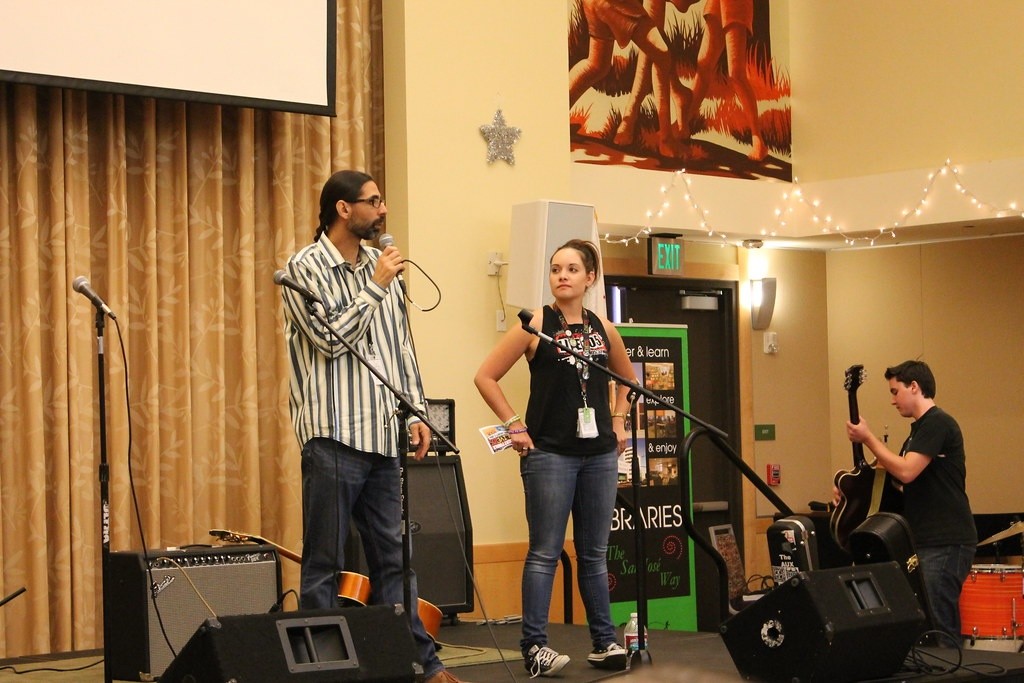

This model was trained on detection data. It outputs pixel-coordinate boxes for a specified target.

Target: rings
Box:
[523,447,528,450]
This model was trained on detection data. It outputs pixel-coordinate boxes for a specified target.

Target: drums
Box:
[957,562,1024,640]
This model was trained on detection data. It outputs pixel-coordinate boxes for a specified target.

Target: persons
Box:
[474,239,635,676]
[283,170,461,683]
[847,360,979,646]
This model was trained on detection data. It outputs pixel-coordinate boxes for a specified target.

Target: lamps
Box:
[749,278,776,330]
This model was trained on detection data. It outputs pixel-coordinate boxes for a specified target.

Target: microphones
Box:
[272,270,323,304]
[378,233,407,294]
[72,275,116,319]
[269,592,286,613]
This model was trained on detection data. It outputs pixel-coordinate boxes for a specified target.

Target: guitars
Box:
[827,362,904,556]
[208,528,445,643]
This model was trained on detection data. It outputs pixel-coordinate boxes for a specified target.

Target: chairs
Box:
[709,523,772,615]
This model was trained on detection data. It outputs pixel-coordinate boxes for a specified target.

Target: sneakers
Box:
[586,641,628,667]
[524,642,571,680]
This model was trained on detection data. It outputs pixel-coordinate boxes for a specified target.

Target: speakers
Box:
[505,200,594,310]
[750,278,777,330]
[339,456,474,624]
[157,603,425,683]
[108,544,284,683]
[719,561,927,683]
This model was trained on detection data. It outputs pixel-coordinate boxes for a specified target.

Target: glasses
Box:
[351,196,385,208]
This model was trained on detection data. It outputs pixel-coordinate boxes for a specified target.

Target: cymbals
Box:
[976,521,1024,546]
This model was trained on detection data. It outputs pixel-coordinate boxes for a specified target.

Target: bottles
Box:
[624,612,648,669]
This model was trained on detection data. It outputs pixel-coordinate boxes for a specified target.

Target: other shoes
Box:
[419,668,469,683]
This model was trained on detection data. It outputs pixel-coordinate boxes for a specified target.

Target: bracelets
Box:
[509,423,528,434]
[504,415,521,428]
[612,413,626,422]
